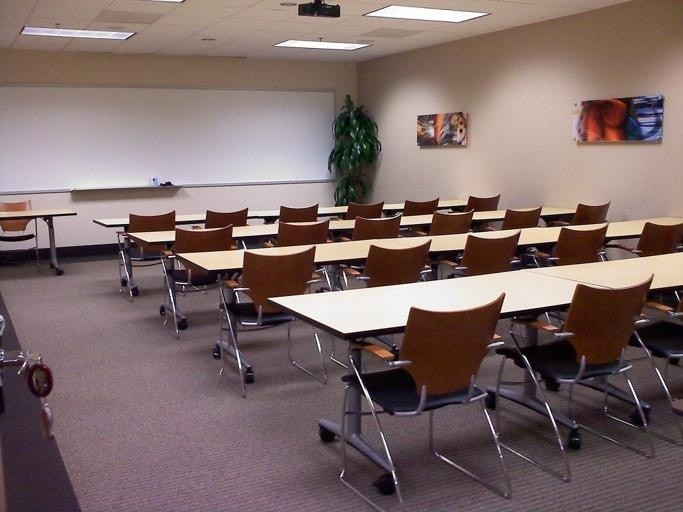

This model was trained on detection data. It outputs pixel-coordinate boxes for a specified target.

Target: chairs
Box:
[600,295,683,450]
[334,292,517,511]
[1,198,41,271]
[492,271,657,484]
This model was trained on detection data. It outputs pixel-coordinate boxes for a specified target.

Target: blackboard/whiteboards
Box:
[1,84,336,194]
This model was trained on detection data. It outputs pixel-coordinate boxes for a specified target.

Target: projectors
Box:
[297,3,340,18]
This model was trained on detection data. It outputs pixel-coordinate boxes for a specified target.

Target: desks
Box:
[0,208,78,278]
[264,252,683,496]
